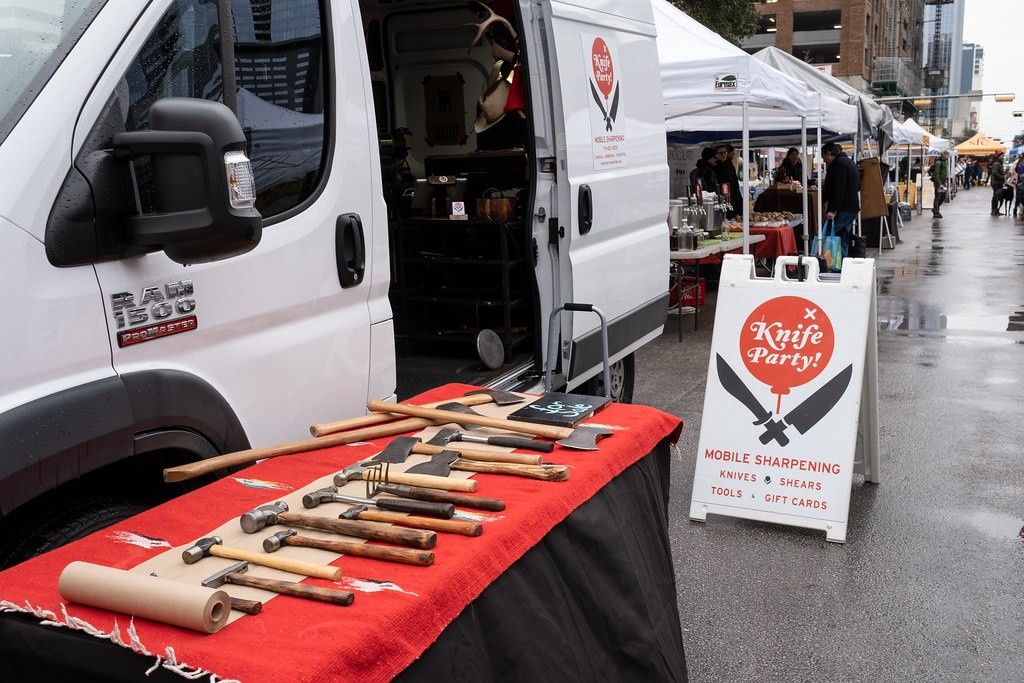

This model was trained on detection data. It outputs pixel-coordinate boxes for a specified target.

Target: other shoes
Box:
[932,208,936,214]
[934,212,943,218]
[991,208,1003,216]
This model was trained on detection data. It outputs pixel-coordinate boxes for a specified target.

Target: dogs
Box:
[994,184,1014,216]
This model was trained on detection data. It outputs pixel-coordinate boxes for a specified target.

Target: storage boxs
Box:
[670,277,705,306]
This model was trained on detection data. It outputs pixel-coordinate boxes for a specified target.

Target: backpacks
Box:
[928,159,943,176]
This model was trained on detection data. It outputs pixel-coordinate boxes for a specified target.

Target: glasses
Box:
[717,151,728,155]
[714,156,718,160]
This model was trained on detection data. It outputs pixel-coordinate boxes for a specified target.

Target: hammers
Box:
[148,571,262,616]
[200,560,354,606]
[302,485,455,519]
[338,504,484,538]
[182,536,343,582]
[262,529,435,568]
[239,501,437,549]
[333,459,478,495]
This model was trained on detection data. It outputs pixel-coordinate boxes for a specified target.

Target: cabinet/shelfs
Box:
[396,213,535,364]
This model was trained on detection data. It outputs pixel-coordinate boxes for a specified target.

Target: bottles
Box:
[677,219,694,252]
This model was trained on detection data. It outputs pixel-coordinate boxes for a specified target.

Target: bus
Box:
[838,144,931,213]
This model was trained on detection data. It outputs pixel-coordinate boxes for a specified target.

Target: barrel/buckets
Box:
[700,201,723,231]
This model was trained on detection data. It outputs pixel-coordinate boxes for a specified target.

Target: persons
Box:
[690,142,1024,261]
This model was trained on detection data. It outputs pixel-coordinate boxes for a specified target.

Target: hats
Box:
[994,150,1002,157]
[701,147,716,161]
[473,77,513,133]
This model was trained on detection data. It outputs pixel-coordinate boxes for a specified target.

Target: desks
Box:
[1,382,688,682]
[730,218,804,277]
[670,235,765,342]
[753,189,828,250]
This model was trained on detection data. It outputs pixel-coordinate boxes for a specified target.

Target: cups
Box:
[697,233,704,249]
[721,226,729,241]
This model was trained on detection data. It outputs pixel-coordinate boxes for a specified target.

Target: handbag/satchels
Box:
[811,218,842,272]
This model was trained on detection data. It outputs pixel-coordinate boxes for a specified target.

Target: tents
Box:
[650,0,1006,274]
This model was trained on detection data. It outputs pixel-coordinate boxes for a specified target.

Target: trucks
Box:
[0,0,670,683]
